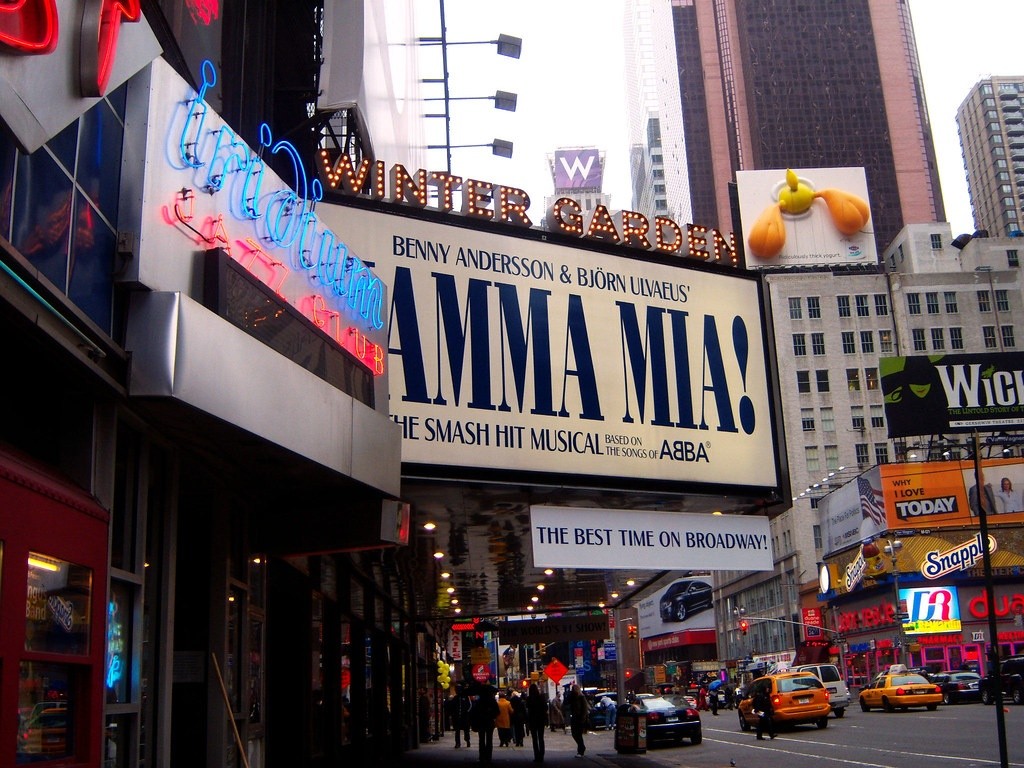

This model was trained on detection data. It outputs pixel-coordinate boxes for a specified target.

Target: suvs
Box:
[978,654,1024,705]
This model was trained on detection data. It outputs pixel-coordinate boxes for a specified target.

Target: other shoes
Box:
[500,741,524,747]
[454,742,460,748]
[467,741,470,747]
[575,754,584,757]
[757,736,765,740]
[771,734,777,739]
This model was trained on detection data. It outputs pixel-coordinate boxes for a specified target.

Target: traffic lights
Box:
[627,625,632,640]
[632,626,637,638]
[740,621,749,629]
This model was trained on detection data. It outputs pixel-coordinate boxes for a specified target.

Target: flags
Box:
[857,476,886,526]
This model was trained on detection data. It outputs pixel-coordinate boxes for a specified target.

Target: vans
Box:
[786,664,852,718]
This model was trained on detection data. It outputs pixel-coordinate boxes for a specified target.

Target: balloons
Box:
[436,660,450,689]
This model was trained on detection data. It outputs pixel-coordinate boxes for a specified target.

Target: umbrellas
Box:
[708,679,721,690]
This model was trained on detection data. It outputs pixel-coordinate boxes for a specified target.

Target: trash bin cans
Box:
[615,704,646,755]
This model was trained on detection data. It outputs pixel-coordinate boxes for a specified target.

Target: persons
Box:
[988,478,1022,513]
[600,695,616,730]
[751,685,777,740]
[723,687,733,711]
[969,471,998,516]
[503,644,518,671]
[710,689,719,715]
[696,685,709,712]
[550,684,594,758]
[448,683,549,761]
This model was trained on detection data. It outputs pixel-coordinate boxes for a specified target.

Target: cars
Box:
[578,687,654,731]
[738,671,832,733]
[908,666,983,705]
[659,580,714,623]
[858,663,943,713]
[637,694,703,748]
